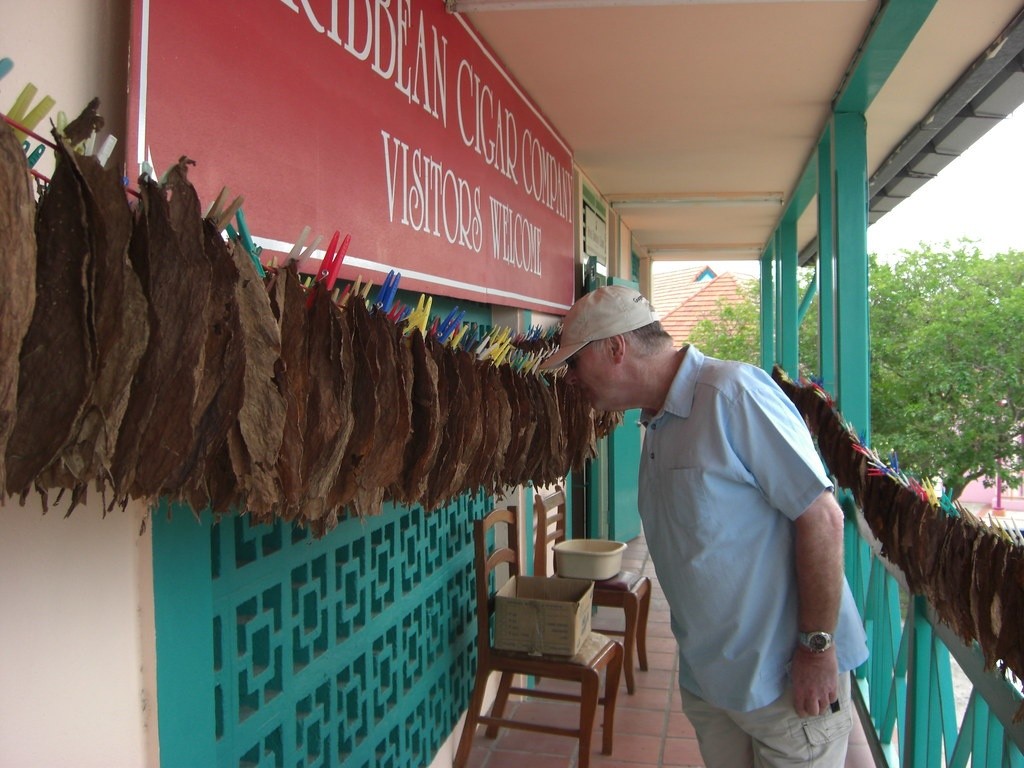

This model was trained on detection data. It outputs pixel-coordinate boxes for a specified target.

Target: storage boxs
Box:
[494,575,596,656]
[552,540,628,581]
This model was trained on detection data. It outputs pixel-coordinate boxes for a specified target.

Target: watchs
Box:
[797,630,834,653]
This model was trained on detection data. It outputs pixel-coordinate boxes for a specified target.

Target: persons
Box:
[537,286,869,768]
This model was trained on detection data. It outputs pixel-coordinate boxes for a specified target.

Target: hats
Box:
[538,285,657,368]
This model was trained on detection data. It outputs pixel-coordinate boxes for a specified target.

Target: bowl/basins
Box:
[551,539,627,581]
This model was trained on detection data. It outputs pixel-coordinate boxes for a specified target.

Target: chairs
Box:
[452,505,625,768]
[533,485,652,695]
